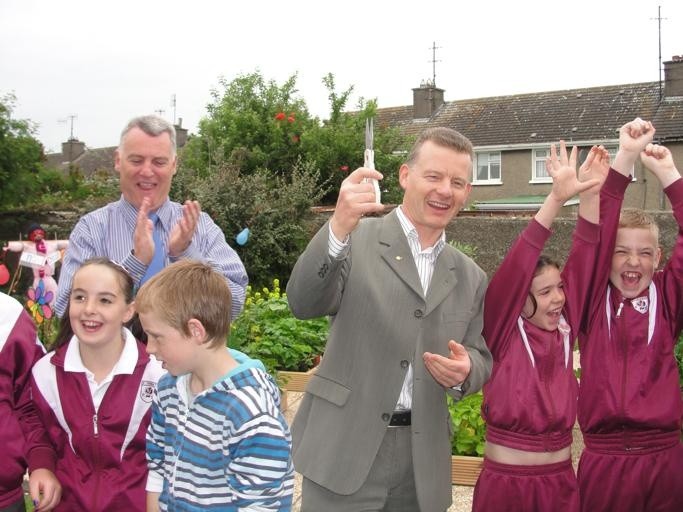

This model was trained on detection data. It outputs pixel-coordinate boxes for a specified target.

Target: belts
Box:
[385,406,412,428]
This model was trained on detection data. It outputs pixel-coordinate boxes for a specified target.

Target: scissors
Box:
[359,116,381,204]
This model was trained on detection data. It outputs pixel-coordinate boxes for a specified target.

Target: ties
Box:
[139,210,166,291]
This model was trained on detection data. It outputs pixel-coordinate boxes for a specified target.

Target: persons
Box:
[286,126,495,512]
[470,140,611,512]
[133,259,295,511]
[53,115,248,345]
[1,289,64,511]
[30,257,155,512]
[575,117,682,511]
